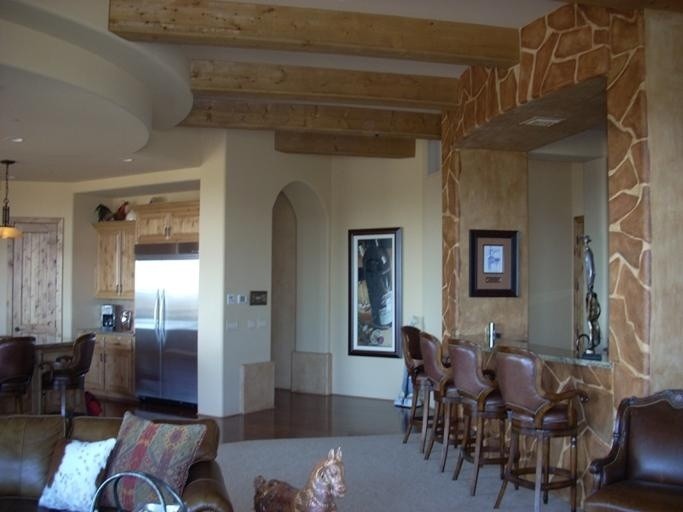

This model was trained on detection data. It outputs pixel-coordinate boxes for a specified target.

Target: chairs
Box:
[0,333,96,417]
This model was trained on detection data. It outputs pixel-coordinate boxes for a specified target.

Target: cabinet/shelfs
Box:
[91,221,135,300]
[84,333,140,406]
[132,200,200,244]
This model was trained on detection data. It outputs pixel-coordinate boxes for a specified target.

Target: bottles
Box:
[361,239,392,330]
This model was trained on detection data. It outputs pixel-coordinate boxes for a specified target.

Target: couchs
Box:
[0,414,234,512]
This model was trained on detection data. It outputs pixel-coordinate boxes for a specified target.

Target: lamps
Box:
[0,160,22,240]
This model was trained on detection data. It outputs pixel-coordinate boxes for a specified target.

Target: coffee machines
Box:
[99,304,116,333]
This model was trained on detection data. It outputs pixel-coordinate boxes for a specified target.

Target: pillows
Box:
[38,411,208,512]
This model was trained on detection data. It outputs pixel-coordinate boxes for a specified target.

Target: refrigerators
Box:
[134,255,198,406]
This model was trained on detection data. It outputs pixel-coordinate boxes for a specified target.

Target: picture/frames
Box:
[348,227,402,359]
[469,230,519,297]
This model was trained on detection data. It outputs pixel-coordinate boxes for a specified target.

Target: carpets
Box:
[215,432,581,512]
